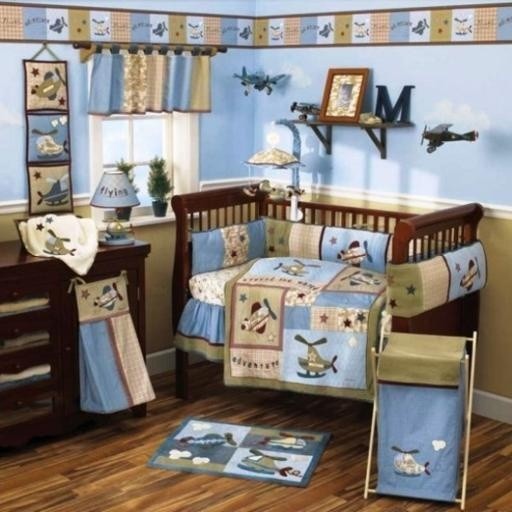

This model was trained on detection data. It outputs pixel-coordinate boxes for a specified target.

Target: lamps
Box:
[89,170,141,246]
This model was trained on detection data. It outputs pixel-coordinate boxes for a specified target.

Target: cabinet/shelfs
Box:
[0,234,151,459]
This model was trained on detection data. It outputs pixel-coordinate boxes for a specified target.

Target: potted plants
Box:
[147,154,174,217]
[114,157,140,220]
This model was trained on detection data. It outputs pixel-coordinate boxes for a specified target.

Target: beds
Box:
[171,184,483,401]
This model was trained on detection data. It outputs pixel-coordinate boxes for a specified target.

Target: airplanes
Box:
[235,65,287,98]
[290,102,323,121]
[421,121,479,155]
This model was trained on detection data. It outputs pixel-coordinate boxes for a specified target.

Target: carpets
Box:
[148,416,332,489]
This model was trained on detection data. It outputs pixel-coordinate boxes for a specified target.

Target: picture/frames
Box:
[320,68,369,122]
